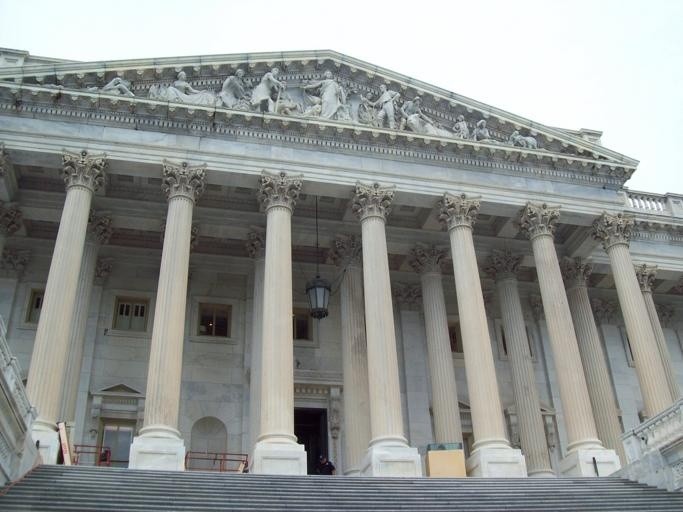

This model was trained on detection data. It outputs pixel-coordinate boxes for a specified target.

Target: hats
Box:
[319,454,325,460]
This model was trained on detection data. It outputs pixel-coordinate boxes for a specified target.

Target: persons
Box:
[313,454,336,474]
[100,67,523,147]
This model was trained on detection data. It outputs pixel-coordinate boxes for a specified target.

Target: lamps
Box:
[304,195,331,320]
